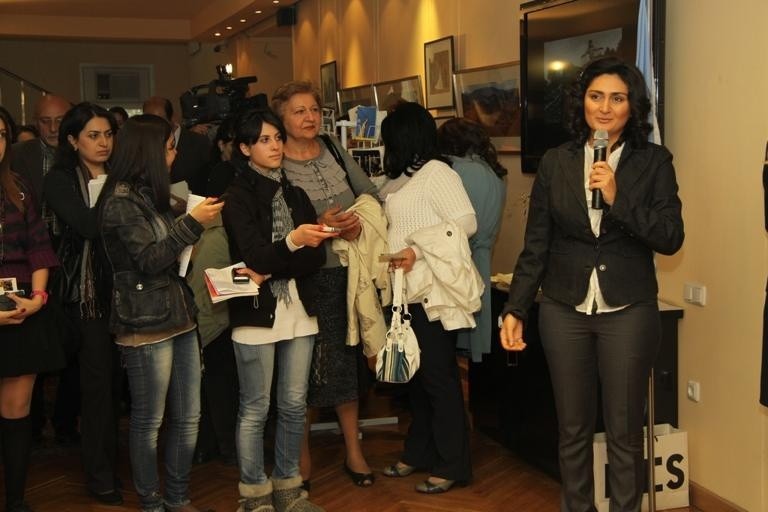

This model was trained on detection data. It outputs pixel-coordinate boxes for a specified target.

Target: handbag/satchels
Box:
[108,269,198,336]
[56,233,86,305]
[592,423,689,511]
[376,321,421,383]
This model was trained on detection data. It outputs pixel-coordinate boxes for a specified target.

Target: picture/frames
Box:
[424,35,455,110]
[320,60,338,112]
[519,0,665,173]
[372,75,424,115]
[452,62,521,154]
[337,83,374,117]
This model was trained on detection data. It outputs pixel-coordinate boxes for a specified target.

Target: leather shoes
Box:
[341,454,457,494]
[96,486,124,505]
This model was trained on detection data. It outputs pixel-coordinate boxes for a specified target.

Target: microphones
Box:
[591,128,609,210]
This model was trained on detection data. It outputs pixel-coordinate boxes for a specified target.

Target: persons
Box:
[2,79,382,512]
[499,57,686,512]
[378,101,509,493]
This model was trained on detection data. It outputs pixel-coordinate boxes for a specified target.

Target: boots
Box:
[238,476,325,512]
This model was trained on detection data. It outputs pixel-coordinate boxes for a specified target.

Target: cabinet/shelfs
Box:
[468,273,684,486]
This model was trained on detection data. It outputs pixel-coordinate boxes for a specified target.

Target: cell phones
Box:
[232,267,249,283]
[322,225,343,233]
[211,191,229,205]
